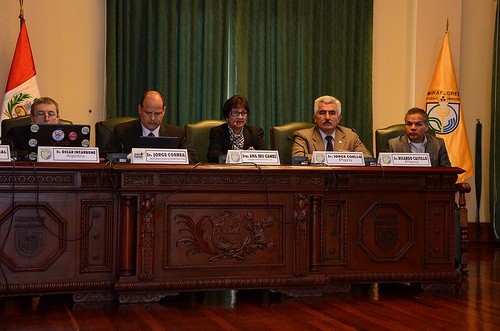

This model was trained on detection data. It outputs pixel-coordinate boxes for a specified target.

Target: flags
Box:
[0,17,43,144]
[422,31,474,184]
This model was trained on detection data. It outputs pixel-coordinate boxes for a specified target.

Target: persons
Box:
[291,96,372,164]
[109,91,200,164]
[7,97,59,158]
[208,95,266,165]
[386,108,452,167]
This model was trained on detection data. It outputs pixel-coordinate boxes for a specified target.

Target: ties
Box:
[325,136,333,151]
[148,132,155,137]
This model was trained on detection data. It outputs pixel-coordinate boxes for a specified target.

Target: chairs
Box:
[270,122,316,163]
[375,124,436,159]
[186,120,227,162]
[1,114,73,139]
[95,117,140,149]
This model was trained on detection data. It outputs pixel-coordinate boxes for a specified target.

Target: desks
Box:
[0,163,466,311]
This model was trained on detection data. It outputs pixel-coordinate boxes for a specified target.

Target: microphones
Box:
[352,128,377,165]
[287,136,308,164]
[0,137,19,157]
[100,121,128,163]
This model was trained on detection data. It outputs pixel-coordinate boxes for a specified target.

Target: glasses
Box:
[230,110,247,116]
[32,112,55,118]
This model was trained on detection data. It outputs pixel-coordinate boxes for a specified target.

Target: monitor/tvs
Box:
[139,136,180,149]
[28,123,91,162]
